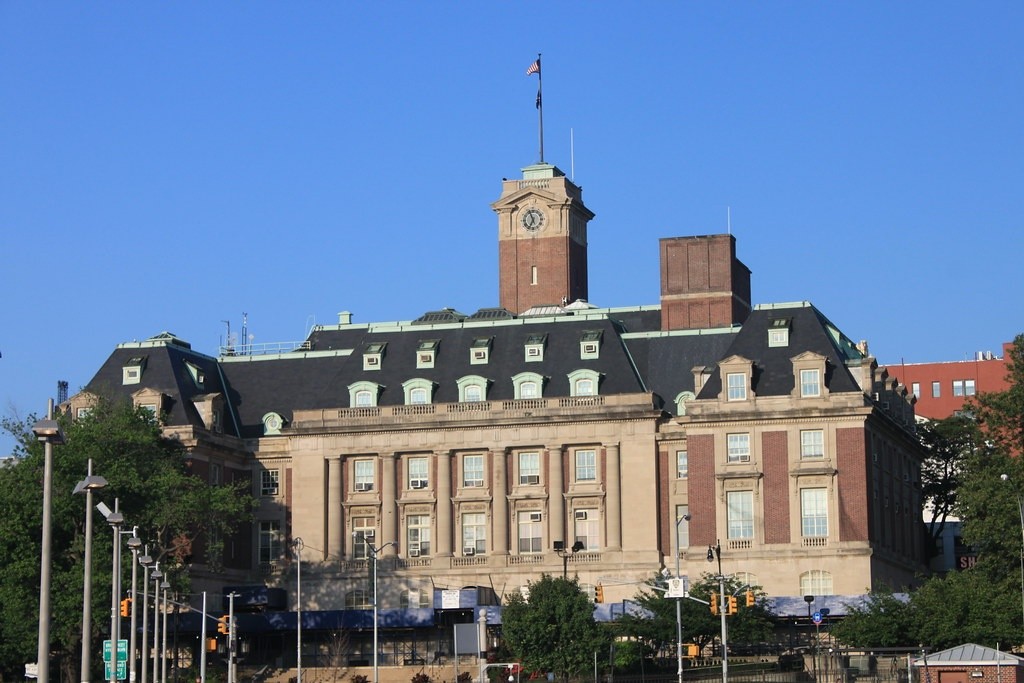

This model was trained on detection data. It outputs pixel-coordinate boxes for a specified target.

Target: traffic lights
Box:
[225,616,230,633]
[746,591,755,608]
[593,586,604,604]
[727,595,738,616]
[120,599,127,616]
[708,594,717,614]
[128,599,133,616]
[218,616,225,633]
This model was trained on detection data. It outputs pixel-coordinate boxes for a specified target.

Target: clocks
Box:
[520,207,547,232]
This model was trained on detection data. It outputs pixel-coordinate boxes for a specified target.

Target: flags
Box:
[526,59,539,75]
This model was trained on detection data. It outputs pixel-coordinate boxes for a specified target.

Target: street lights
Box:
[106,497,126,683]
[707,539,730,683]
[72,457,111,682]
[674,514,690,681]
[31,397,66,682]
[804,594,813,652]
[127,526,171,683]
[352,532,398,682]
[810,635,817,683]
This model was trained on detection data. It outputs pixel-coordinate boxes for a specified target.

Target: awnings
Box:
[262,606,437,629]
[473,593,918,625]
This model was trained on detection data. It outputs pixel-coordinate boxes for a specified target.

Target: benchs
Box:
[404,659,425,666]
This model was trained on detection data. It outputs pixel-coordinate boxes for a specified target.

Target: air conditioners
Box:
[367,358,377,364]
[529,348,539,355]
[355,483,367,490]
[364,530,375,536]
[475,480,484,487]
[584,343,596,352]
[462,547,474,554]
[409,549,420,557]
[410,479,423,488]
[576,511,586,520]
[422,355,431,362]
[527,475,539,484]
[474,352,485,359]
[739,455,749,462]
[198,376,206,382]
[529,513,541,521]
